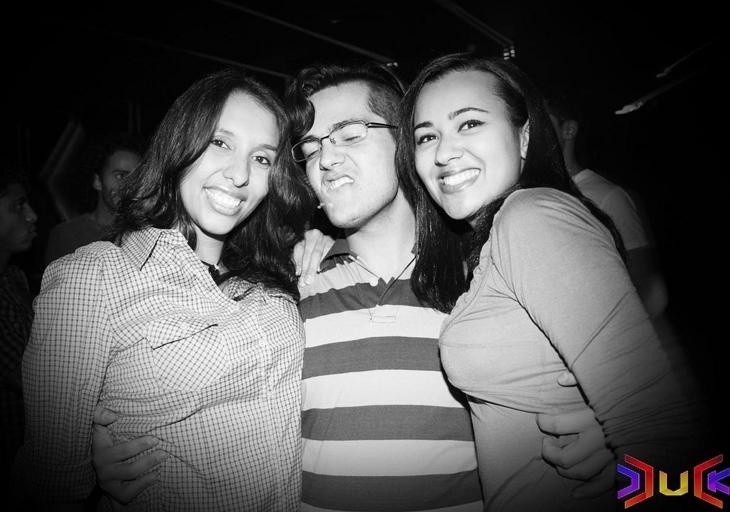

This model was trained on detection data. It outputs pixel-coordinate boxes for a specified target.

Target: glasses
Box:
[290,119,398,162]
